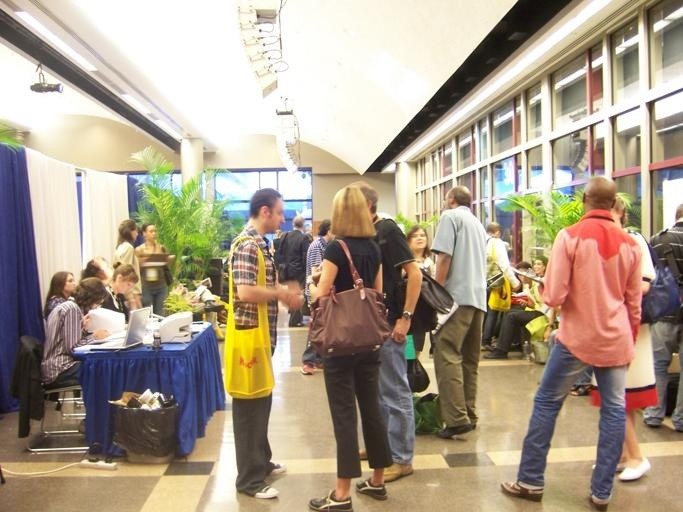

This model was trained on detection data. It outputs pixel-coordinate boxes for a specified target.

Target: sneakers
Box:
[593,455,651,481]
[268,460,286,479]
[355,477,388,501]
[501,479,544,504]
[589,492,612,511]
[254,485,280,499]
[300,363,315,376]
[482,349,508,360]
[308,489,354,511]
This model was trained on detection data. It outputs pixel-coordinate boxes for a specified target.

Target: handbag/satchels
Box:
[223,236,276,401]
[394,266,453,335]
[637,228,680,327]
[308,240,391,359]
[413,392,445,436]
[488,243,513,312]
[277,232,290,283]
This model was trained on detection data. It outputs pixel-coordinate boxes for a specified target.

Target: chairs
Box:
[8,335,90,452]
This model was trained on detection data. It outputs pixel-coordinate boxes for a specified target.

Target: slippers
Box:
[383,460,414,484]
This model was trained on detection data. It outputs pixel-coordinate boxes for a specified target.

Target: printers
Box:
[157,311,193,343]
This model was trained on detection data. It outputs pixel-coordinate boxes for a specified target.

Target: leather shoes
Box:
[437,420,478,439]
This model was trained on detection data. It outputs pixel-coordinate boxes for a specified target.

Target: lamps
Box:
[30,63,63,93]
[234,1,300,174]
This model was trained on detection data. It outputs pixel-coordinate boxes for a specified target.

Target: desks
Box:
[73,321,226,455]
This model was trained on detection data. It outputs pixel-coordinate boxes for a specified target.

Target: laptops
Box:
[90,307,151,351]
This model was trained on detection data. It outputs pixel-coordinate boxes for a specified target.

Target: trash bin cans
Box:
[115,401,178,464]
